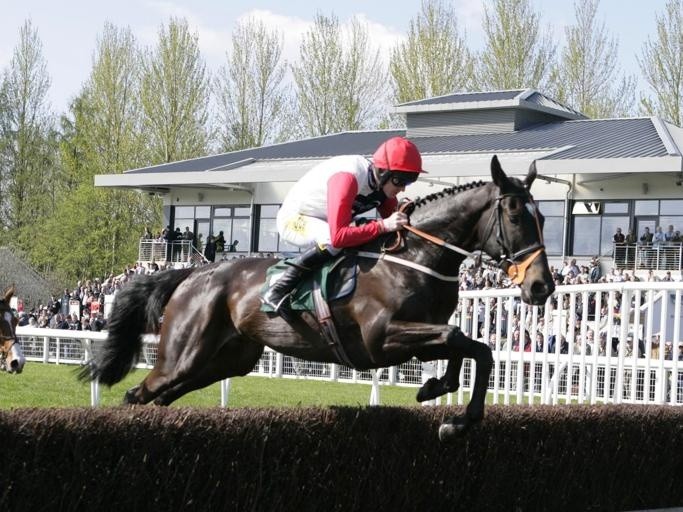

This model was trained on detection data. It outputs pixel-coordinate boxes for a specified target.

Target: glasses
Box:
[384,142,406,186]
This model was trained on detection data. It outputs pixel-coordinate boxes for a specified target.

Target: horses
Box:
[68,155,557,448]
[0,282,26,374]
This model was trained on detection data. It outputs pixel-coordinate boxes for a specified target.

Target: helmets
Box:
[373,138,429,174]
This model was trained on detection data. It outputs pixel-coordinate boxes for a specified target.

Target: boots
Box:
[260,244,326,314]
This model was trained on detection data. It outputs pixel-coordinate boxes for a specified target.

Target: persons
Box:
[263,352,426,384]
[11,257,162,360]
[263,136,428,312]
[451,254,682,402]
[143,225,278,271]
[614,225,682,273]
